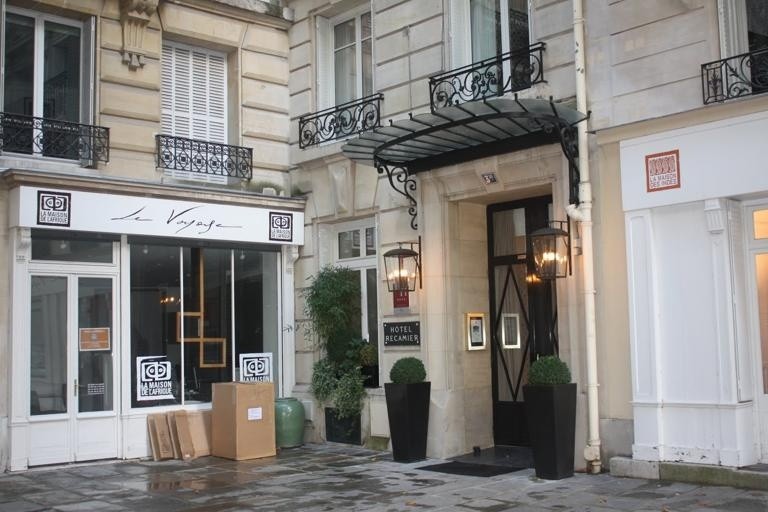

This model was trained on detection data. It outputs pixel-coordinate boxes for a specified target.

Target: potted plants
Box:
[361,344,379,388]
[384,355,431,462]
[279,262,370,446]
[523,355,577,480]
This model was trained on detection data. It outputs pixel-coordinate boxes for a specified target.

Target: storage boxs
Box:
[211,382,276,460]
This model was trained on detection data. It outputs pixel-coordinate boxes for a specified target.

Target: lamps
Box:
[383,236,422,297]
[529,212,573,281]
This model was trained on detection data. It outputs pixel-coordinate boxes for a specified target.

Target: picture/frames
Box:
[466,312,486,350]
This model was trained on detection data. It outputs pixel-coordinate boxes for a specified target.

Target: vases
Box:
[274,397,305,448]
